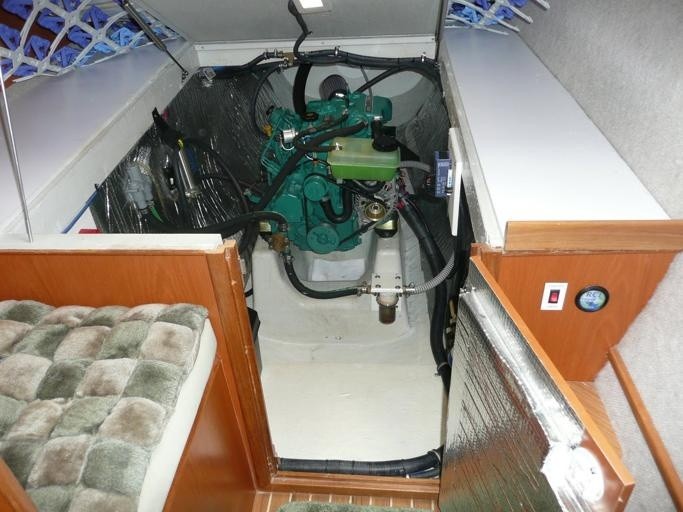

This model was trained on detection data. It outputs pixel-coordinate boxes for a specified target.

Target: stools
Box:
[1,298,258,510]
